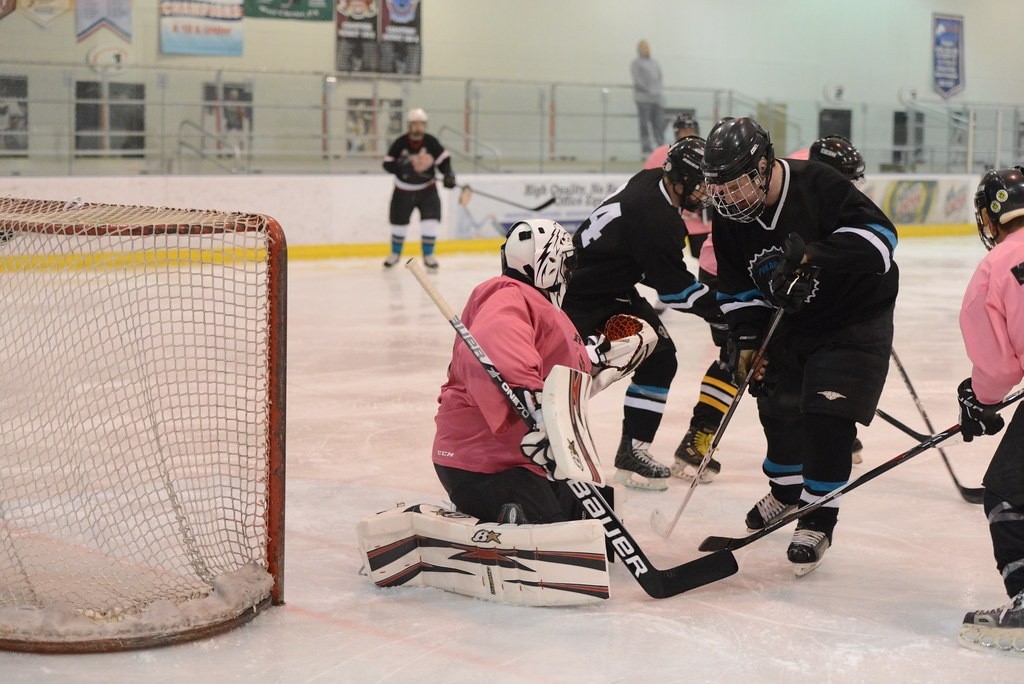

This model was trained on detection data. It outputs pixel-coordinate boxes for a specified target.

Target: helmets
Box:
[500,218,575,306]
[974,165,1024,252]
[700,116,774,224]
[673,113,699,136]
[811,134,869,184]
[662,135,711,214]
[407,108,428,140]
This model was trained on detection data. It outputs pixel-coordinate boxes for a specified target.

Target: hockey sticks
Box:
[404,255,741,601]
[697,387,1024,553]
[889,346,987,505]
[432,176,556,212]
[648,241,820,535]
[873,407,965,448]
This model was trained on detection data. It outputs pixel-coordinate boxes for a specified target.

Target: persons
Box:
[224,87,247,170]
[560,135,707,490]
[957,165,1024,657]
[432,218,593,524]
[631,41,666,153]
[644,113,712,260]
[670,134,866,484]
[382,108,456,268]
[701,117,900,575]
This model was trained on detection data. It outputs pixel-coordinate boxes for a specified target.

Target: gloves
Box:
[747,245,820,315]
[445,170,455,188]
[732,323,785,401]
[958,377,1005,442]
[705,290,766,325]
[395,156,414,179]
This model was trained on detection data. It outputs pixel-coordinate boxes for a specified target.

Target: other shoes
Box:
[424,253,438,274]
[851,437,864,464]
[384,252,400,267]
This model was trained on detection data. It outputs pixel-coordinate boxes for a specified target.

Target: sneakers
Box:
[669,422,722,484]
[613,418,671,489]
[958,587,1024,658]
[744,475,805,534]
[788,485,843,578]
